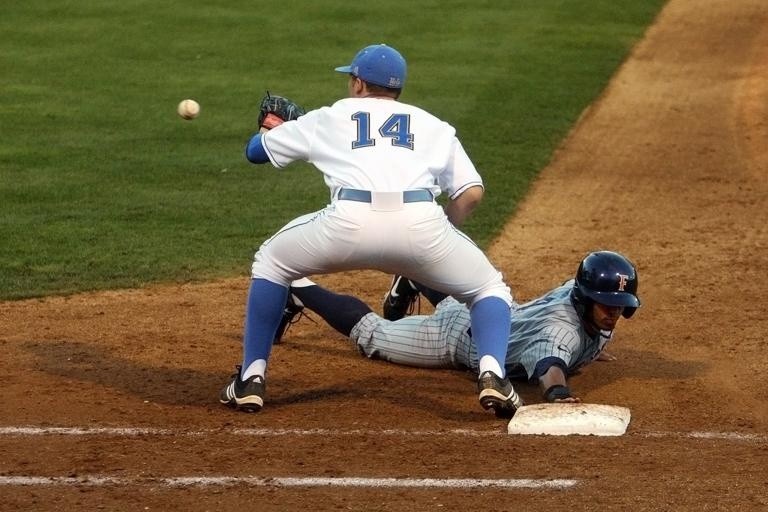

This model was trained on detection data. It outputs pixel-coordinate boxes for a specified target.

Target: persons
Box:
[272,251,640,404]
[219,43,524,418]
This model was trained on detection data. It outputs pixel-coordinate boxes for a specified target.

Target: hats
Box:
[334,43,407,89]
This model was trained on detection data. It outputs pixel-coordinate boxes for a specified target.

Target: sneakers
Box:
[219,368,267,412]
[383,274,419,321]
[272,292,304,345]
[477,371,523,418]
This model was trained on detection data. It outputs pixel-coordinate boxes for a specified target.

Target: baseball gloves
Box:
[258,92,306,131]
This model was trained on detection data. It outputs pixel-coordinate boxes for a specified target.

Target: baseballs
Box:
[177,98,201,119]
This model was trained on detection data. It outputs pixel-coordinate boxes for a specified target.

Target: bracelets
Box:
[544,384,571,400]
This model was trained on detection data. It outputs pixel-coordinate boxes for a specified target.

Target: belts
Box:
[338,189,434,203]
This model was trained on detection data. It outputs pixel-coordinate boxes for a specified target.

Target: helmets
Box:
[573,251,641,319]
